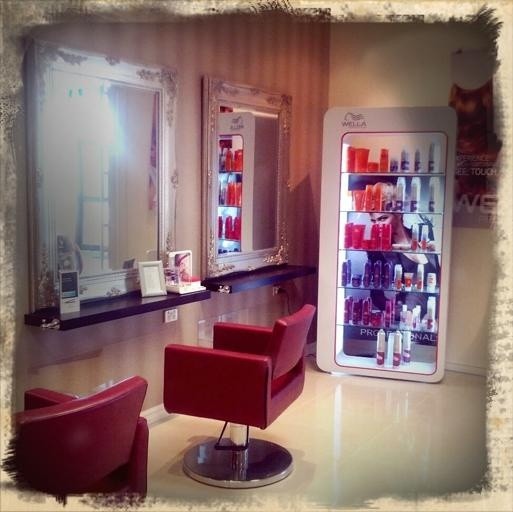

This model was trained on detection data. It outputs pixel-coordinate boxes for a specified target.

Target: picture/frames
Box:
[137,260,167,298]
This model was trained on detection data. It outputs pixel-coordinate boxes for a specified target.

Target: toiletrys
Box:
[217,146,246,255]
[340,144,443,370]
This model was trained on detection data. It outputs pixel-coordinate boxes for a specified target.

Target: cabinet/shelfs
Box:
[315,104,461,385]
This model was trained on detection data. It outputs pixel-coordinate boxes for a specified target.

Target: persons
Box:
[177,263,189,283]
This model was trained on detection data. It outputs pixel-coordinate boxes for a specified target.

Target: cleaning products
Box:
[217,149,242,240]
[341,141,440,368]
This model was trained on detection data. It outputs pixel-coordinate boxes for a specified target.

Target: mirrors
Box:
[25,38,172,314]
[201,75,292,282]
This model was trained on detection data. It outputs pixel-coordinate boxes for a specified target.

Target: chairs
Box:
[3,374,152,508]
[162,303,316,490]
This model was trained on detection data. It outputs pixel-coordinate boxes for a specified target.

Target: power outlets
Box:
[164,308,178,323]
[273,286,283,296]
